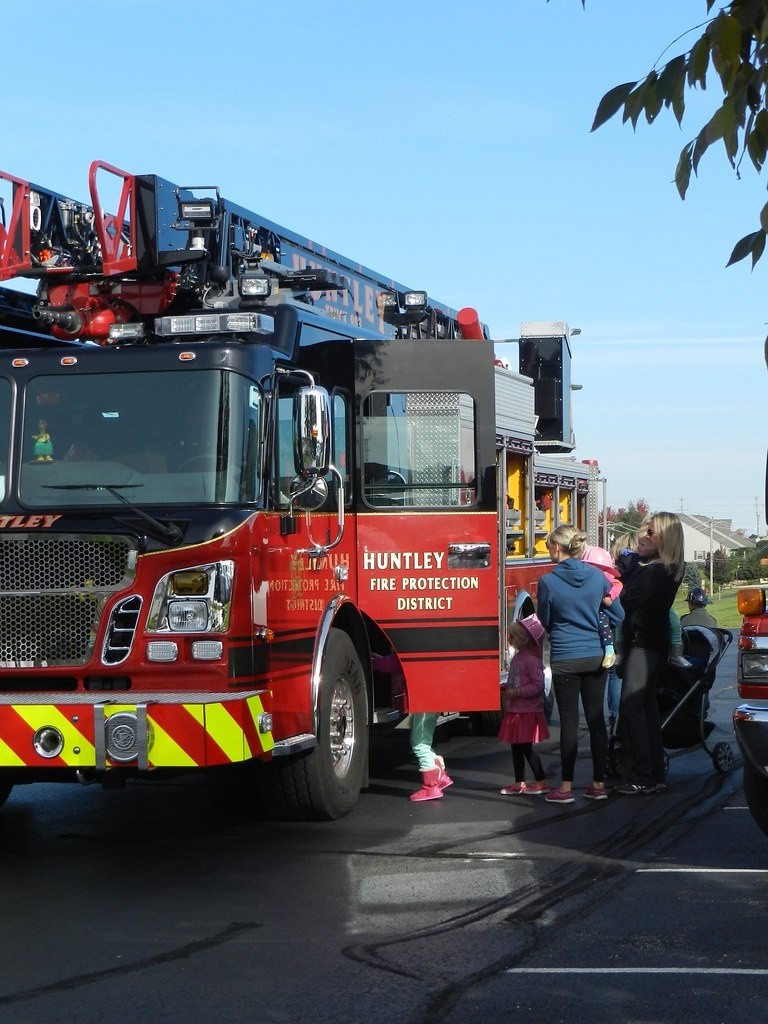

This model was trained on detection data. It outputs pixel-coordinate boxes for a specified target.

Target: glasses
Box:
[545,541,550,550]
[646,529,659,537]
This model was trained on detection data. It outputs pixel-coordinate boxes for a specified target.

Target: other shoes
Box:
[500,784,529,794]
[602,652,617,668]
[524,784,554,794]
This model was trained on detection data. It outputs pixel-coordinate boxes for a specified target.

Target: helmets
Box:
[684,588,717,604]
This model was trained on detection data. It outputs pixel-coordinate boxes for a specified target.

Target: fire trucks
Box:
[1,157,611,826]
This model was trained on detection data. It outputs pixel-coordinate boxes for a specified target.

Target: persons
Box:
[501,614,551,793]
[371,653,453,801]
[615,512,693,794]
[680,588,717,628]
[537,525,625,803]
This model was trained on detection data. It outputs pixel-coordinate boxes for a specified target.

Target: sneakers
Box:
[615,782,658,794]
[544,789,577,804]
[583,784,611,799]
[657,780,669,791]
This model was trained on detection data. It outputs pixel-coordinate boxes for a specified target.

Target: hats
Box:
[582,546,623,577]
[516,614,547,648]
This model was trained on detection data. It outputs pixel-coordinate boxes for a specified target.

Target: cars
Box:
[731,585,768,838]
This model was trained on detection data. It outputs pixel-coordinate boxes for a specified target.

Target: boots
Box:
[409,768,444,801]
[434,754,454,792]
[614,642,627,665]
[671,642,695,668]
